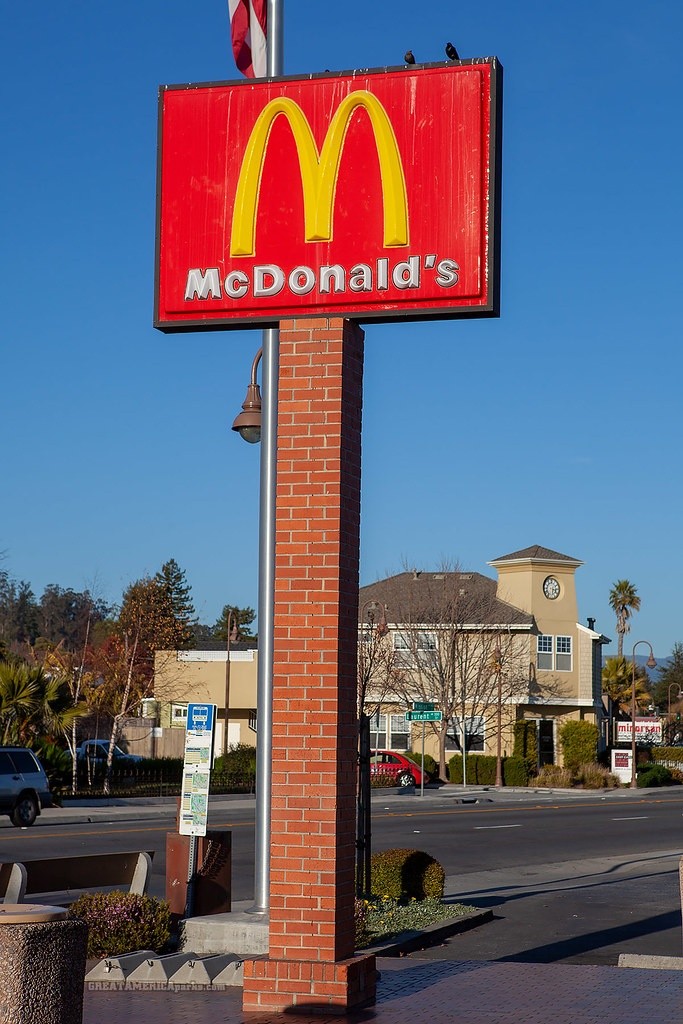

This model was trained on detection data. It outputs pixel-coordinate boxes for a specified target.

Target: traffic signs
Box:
[405,702,440,722]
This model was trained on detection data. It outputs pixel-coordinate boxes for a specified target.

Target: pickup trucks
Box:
[63,739,142,764]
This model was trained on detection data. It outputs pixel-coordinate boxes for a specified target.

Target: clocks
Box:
[544,578,560,599]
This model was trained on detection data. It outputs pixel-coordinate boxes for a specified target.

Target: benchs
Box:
[0,851,157,907]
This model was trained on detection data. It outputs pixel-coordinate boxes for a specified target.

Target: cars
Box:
[370,752,430,787]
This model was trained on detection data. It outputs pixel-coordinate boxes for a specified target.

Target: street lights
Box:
[667,683,683,741]
[361,597,391,717]
[632,639,657,788]
[225,610,243,755]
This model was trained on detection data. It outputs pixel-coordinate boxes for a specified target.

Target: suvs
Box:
[0,745,53,827]
